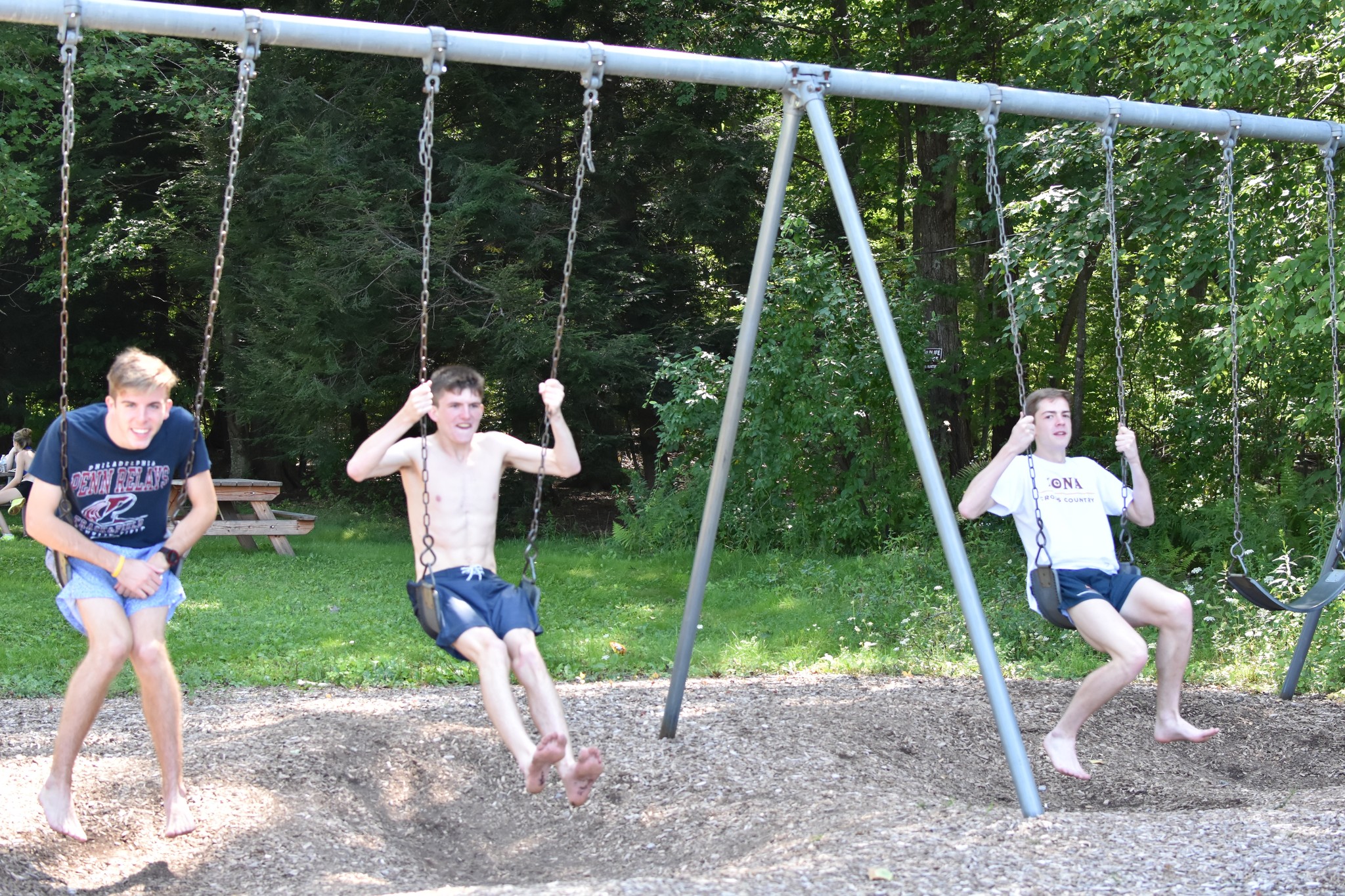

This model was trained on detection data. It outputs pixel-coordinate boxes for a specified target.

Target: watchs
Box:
[158,546,179,566]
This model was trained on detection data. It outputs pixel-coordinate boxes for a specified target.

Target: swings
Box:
[54,23,265,604]
[406,41,601,646]
[1225,125,1345,612]
[972,101,1135,630]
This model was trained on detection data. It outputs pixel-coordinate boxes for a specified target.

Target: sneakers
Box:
[8,498,26,515]
[0,531,15,542]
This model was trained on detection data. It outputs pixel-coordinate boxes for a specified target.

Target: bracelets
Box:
[111,555,124,578]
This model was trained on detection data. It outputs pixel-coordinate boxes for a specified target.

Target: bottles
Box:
[0,455,7,473]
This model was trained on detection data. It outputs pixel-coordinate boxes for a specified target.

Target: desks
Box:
[0,469,30,536]
[165,478,316,562]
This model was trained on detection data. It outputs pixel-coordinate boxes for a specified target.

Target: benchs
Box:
[0,500,13,506]
[270,509,318,522]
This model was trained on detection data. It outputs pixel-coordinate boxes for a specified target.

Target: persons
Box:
[20,342,219,840]
[344,362,603,809]
[0,428,41,540]
[955,380,1223,779]
